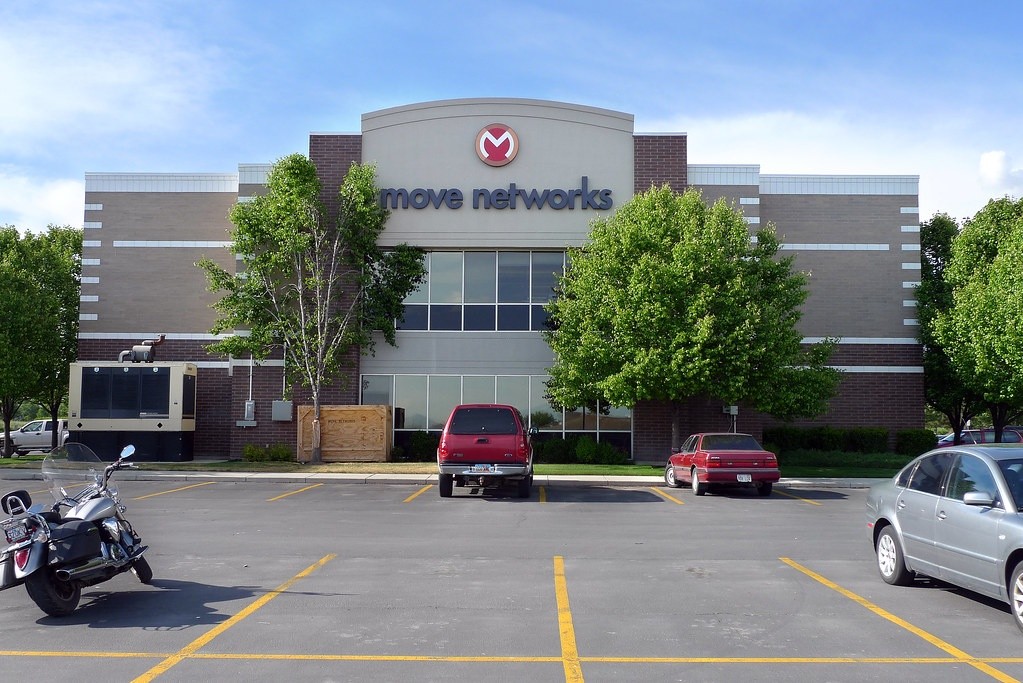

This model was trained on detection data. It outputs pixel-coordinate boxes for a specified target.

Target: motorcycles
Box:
[0,442,153,618]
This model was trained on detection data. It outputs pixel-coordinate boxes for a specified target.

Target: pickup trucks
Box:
[0,418,69,456]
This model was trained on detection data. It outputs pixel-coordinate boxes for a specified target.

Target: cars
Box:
[934,425,1023,448]
[664,433,781,496]
[866,443,1023,636]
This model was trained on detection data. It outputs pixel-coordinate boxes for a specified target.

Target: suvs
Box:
[437,403,539,500]
